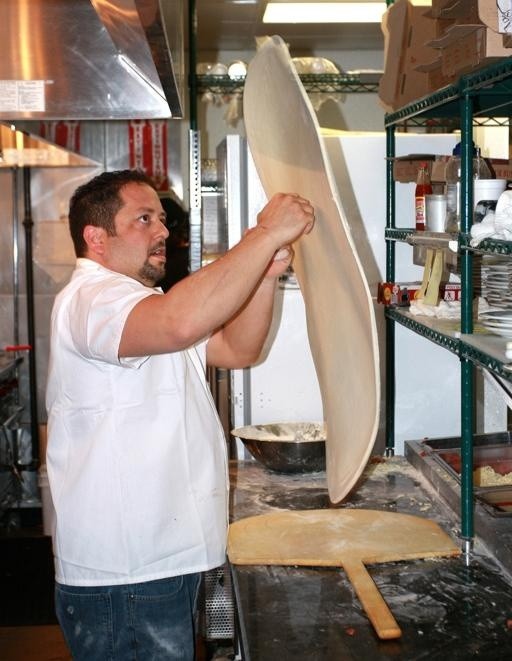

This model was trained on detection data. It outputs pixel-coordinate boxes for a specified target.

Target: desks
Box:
[223,456,511,660]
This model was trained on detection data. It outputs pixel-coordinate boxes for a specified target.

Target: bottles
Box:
[445,140,491,234]
[431,155,446,195]
[414,161,433,232]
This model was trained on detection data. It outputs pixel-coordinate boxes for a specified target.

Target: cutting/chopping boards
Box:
[226,509,464,639]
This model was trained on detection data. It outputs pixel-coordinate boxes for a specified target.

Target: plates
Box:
[479,261,512,338]
[450,255,488,300]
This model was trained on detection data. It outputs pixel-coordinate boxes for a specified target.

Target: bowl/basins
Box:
[230,422,326,473]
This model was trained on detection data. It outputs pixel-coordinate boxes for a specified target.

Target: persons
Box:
[42,167,316,661]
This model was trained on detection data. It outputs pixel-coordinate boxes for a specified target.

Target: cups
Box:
[455,180,508,232]
[422,193,448,234]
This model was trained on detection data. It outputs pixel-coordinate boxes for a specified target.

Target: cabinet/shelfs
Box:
[380,54,511,556]
